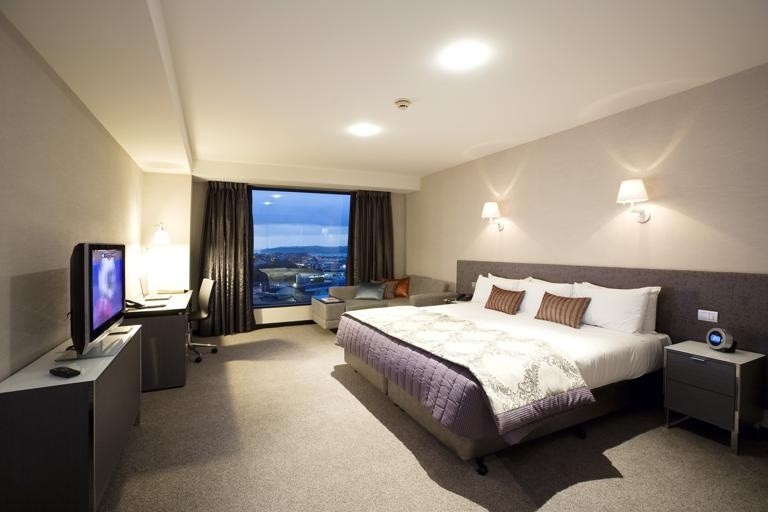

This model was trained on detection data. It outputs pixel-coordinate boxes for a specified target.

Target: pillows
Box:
[485,285,525,315]
[535,291,591,329]
[395,278,410,299]
[581,279,662,334]
[469,274,572,328]
[570,281,650,337]
[370,280,398,299]
[487,272,573,334]
[354,281,386,301]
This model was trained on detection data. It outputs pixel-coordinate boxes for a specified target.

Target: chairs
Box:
[188,278,218,364]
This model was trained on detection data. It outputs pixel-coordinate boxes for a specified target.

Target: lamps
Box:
[616,178,652,224]
[482,201,514,240]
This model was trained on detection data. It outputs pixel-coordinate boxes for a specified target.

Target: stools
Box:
[310,294,346,330]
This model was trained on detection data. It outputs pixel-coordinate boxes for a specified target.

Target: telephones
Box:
[449,293,473,301]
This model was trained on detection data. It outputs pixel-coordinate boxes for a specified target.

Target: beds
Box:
[335,269,673,462]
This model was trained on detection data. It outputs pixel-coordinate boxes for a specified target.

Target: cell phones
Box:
[49,366,80,380]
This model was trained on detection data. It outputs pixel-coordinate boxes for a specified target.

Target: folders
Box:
[155,289,189,294]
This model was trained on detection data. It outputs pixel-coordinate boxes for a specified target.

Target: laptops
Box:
[139,274,172,300]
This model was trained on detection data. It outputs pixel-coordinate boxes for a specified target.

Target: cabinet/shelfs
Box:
[123,291,194,392]
[2,325,144,512]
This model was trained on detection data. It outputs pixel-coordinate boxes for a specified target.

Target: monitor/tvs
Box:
[54,242,126,362]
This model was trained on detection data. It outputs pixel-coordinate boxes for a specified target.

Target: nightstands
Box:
[663,340,767,456]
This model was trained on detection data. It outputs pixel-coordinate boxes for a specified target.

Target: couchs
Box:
[328,274,458,311]
